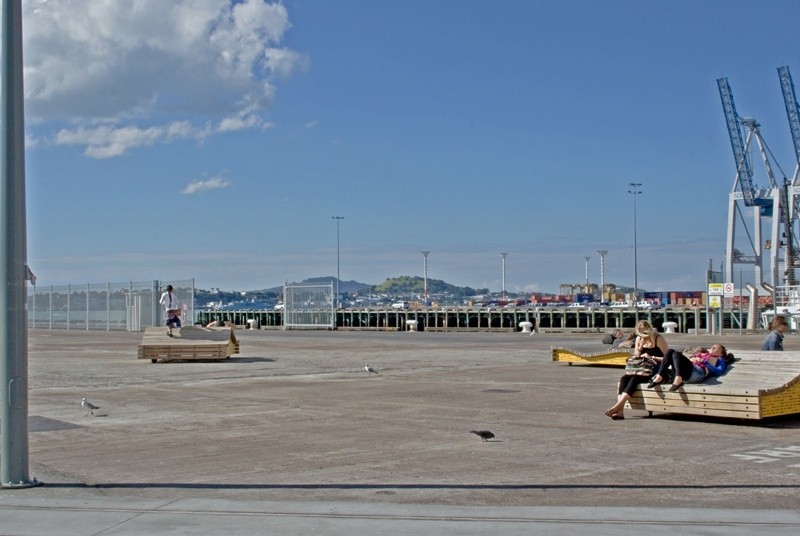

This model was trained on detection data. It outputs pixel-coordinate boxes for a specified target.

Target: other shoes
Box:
[604,407,624,420]
[669,381,684,392]
[647,378,665,388]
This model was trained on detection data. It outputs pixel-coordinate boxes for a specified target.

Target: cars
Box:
[273,299,662,311]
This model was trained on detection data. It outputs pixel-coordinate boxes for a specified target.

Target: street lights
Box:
[597,250,609,303]
[582,256,591,293]
[627,183,642,301]
[421,251,432,303]
[499,252,509,300]
[331,216,344,310]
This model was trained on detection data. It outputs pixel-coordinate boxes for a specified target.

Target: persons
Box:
[605,320,668,420]
[159,285,179,336]
[761,316,788,351]
[647,345,727,391]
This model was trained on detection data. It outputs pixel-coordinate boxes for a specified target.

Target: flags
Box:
[27,267,36,285]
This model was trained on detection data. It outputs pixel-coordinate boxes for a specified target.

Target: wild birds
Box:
[470,428,496,441]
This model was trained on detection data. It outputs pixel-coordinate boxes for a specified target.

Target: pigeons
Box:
[364,363,379,376]
[82,398,100,417]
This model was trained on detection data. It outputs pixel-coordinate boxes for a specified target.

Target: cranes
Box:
[716,65,800,289]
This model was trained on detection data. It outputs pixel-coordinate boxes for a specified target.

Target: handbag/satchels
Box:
[625,356,657,376]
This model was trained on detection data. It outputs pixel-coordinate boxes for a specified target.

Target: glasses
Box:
[781,322,789,326]
